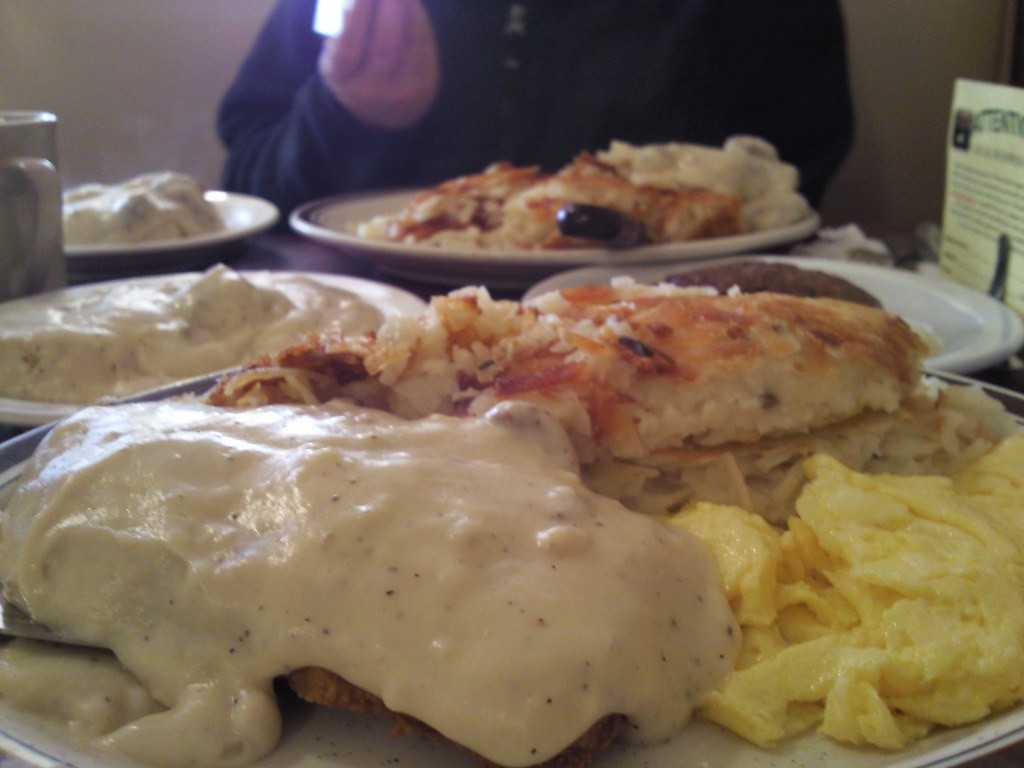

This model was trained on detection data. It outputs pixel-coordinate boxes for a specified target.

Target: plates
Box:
[524,257,1024,382]
[1,362,1021,766]
[290,186,818,288]
[58,189,279,276]
[3,268,428,428]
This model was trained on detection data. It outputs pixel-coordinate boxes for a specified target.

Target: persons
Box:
[209,2,855,219]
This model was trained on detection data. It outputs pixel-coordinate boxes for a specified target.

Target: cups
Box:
[1,109,64,298]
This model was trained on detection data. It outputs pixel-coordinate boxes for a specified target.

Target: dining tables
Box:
[0,231,1024,766]
